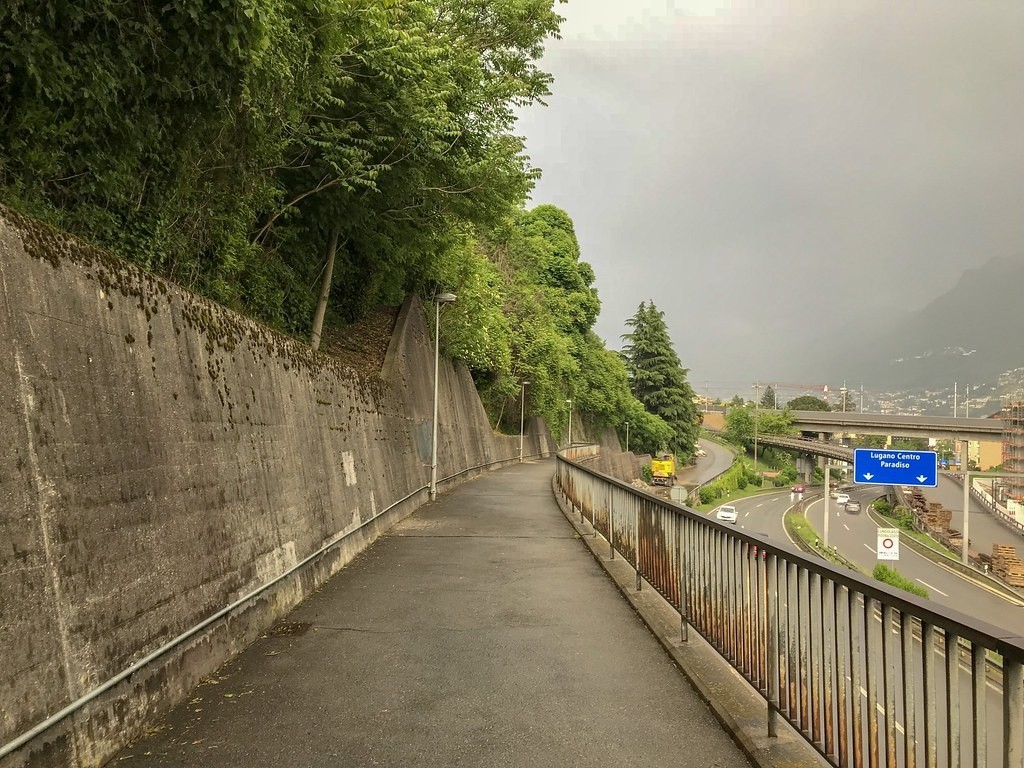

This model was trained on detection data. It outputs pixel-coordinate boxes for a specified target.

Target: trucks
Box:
[650,454,675,486]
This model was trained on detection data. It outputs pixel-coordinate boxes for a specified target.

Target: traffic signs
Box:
[853,449,938,488]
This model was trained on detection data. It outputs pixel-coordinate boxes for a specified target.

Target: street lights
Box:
[565,400,571,445]
[429,293,458,501]
[520,382,531,462]
[624,422,628,452]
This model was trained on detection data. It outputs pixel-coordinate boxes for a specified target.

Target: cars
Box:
[791,484,805,493]
[716,505,739,524]
[845,500,861,513]
[836,494,850,505]
[747,543,766,560]
[831,490,842,499]
[829,480,839,488]
